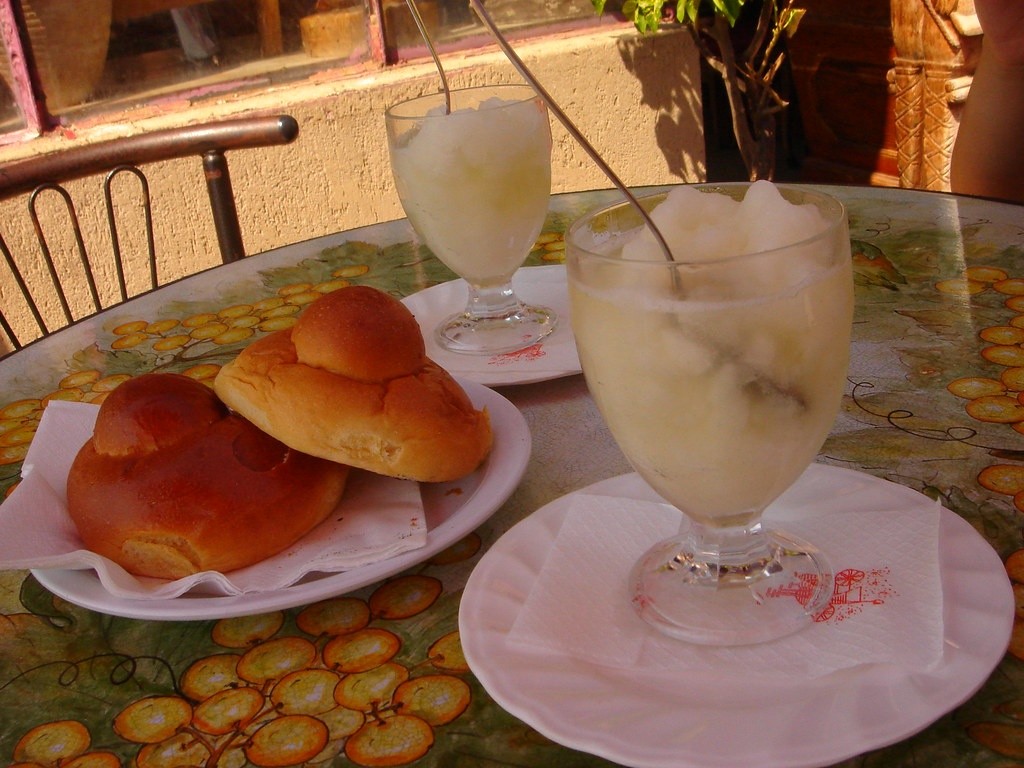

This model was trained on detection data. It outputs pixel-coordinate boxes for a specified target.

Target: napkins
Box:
[424,286,583,373]
[0,399,429,601]
[502,493,948,688]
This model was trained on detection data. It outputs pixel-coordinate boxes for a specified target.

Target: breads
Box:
[64,372,350,578]
[212,282,494,484]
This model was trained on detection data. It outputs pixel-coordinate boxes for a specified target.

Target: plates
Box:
[457,465,1015,768]
[28,376,532,621]
[398,264,583,387]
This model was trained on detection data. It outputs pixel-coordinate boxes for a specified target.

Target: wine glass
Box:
[563,179,856,646]
[384,84,558,356]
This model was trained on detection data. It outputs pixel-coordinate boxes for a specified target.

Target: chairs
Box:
[0,116,298,350]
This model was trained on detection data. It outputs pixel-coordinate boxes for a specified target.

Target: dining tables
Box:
[0,184,1024,768]
